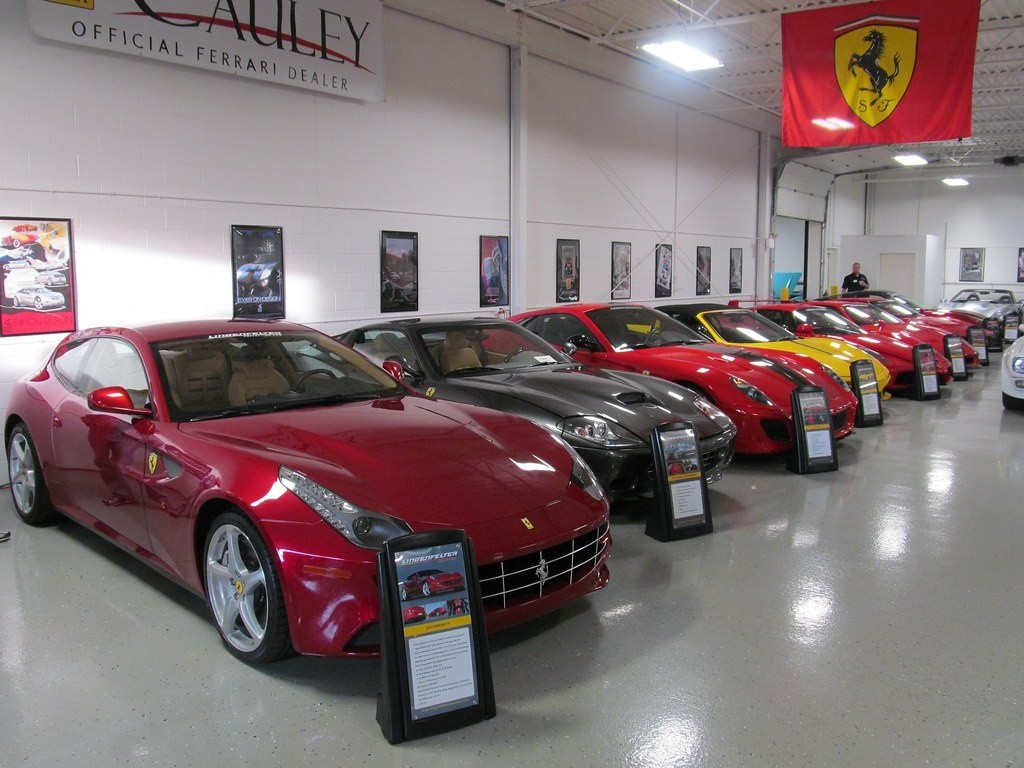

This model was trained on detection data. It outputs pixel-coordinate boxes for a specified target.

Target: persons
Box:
[841,262,870,294]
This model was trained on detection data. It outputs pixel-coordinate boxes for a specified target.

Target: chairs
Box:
[83,341,121,393]
[1000,295,1011,303]
[373,332,418,370]
[120,352,178,411]
[230,346,290,406]
[967,293,979,301]
[544,316,569,340]
[439,329,483,375]
[175,343,230,412]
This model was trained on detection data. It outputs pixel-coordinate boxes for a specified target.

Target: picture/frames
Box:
[611,241,632,299]
[379,229,419,313]
[958,248,986,281]
[555,237,581,303]
[655,243,674,298]
[696,245,712,295]
[1016,248,1024,282]
[479,234,510,307]
[729,248,743,293]
[231,224,287,321]
[0,215,78,336]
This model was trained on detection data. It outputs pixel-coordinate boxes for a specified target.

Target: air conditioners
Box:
[890,151,929,167]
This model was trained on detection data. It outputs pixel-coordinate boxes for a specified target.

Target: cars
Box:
[804,402,825,413]
[428,607,446,618]
[4,317,613,665]
[402,569,464,601]
[664,443,695,460]
[35,271,67,286]
[1001,324,1024,412]
[13,287,64,309]
[403,606,425,624]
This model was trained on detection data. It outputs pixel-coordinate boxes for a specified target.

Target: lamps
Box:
[635,33,725,70]
[943,177,970,186]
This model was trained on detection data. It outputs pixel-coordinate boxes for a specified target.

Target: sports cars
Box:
[799,300,979,367]
[627,303,892,401]
[238,254,280,289]
[831,298,993,347]
[934,288,1024,328]
[814,290,986,326]
[290,316,737,499]
[720,299,952,389]
[481,303,858,456]
